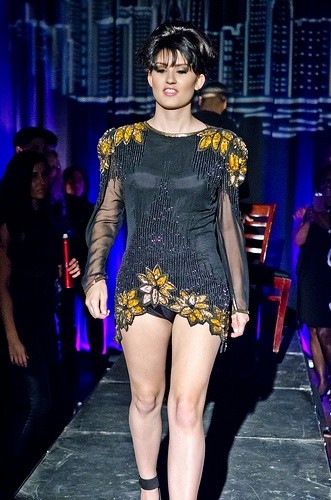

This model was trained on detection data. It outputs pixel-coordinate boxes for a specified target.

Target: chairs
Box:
[239,203,291,353]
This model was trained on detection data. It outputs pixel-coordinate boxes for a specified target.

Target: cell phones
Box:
[314,193,325,212]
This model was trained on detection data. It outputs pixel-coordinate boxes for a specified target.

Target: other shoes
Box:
[139,476,162,500]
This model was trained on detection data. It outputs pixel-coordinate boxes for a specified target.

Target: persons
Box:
[295,182,331,395]
[81,22,250,500]
[0,125,98,438]
[190,80,255,224]
